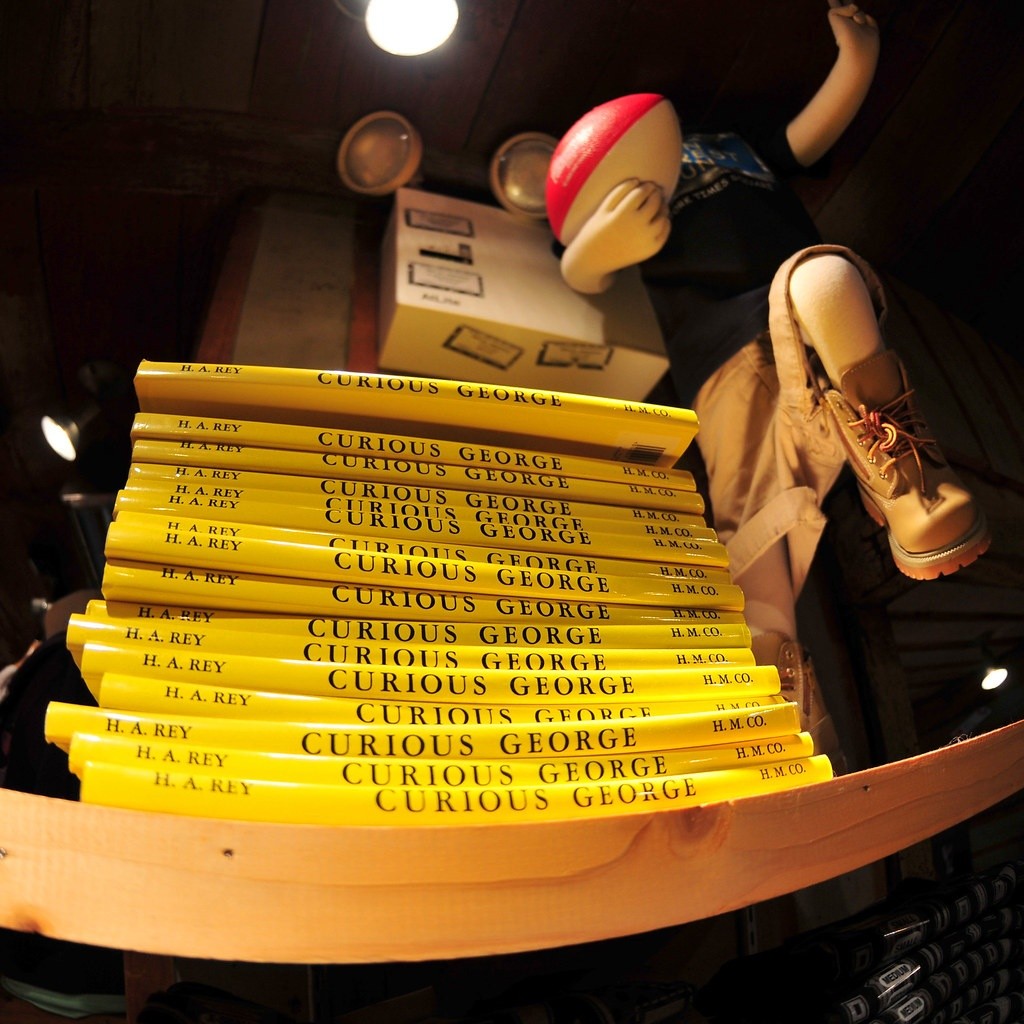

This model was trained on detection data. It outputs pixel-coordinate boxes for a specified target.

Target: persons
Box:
[560,1,991,775]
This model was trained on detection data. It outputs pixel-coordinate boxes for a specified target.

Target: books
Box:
[0,969,126,1019]
[46,360,835,829]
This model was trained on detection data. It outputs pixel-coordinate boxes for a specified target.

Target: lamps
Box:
[39,401,102,461]
[362,0,460,55]
[336,111,670,401]
[981,648,1008,690]
